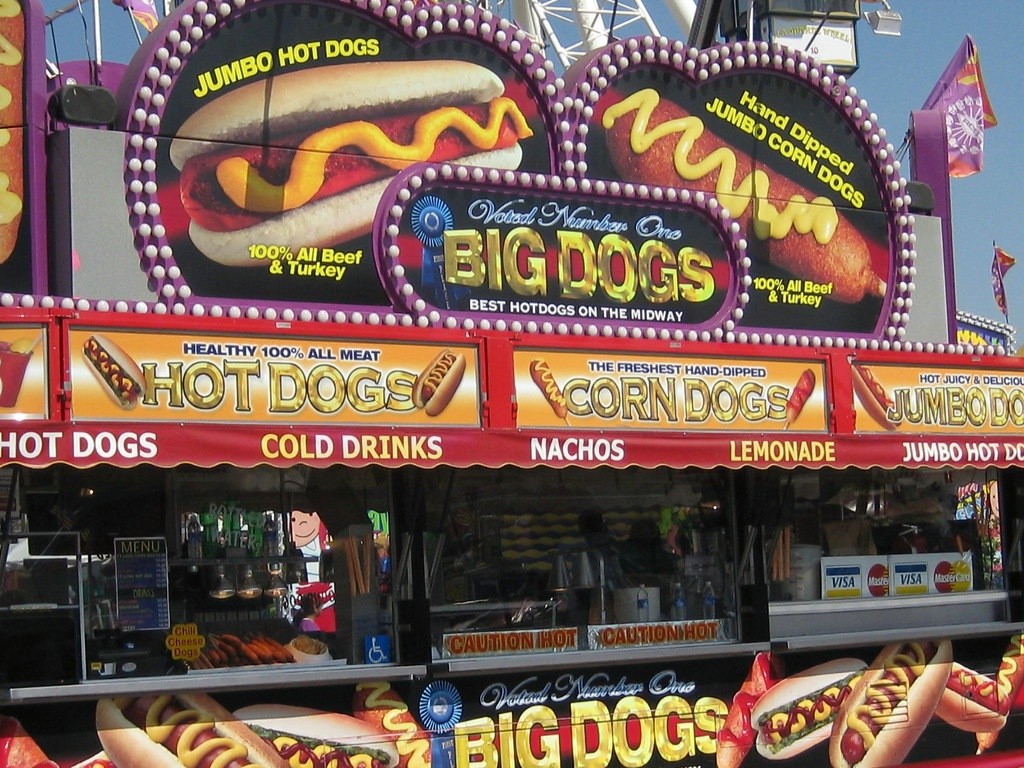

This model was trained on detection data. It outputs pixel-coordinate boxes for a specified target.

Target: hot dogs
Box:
[169,59,534,267]
[411,348,466,416]
[749,635,1009,768]
[81,334,147,410]
[95,687,399,768]
[851,364,903,431]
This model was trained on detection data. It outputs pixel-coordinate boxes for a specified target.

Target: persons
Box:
[620,518,670,578]
[896,485,964,555]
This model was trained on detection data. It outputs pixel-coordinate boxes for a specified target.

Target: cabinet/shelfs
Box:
[0,530,85,686]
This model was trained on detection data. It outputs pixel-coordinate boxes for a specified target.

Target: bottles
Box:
[187,496,283,558]
[638,584,650,623]
[673,583,686,621]
[703,582,715,619]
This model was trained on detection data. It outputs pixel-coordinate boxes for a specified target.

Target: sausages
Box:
[352,680,434,768]
[785,369,815,424]
[529,357,568,418]
[602,87,889,304]
[204,634,295,668]
[976,633,1024,756]
[715,651,782,768]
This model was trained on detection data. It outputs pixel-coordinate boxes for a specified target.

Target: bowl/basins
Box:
[287,637,331,662]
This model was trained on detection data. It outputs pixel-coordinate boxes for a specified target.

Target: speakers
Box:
[905,181,934,215]
[48,85,118,125]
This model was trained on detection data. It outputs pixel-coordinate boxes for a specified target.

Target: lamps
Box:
[571,551,595,590]
[237,565,263,599]
[209,565,235,599]
[264,563,288,598]
[547,555,570,592]
[864,0,901,36]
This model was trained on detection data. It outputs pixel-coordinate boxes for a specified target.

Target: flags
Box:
[922,35,998,177]
[991,245,1016,316]
